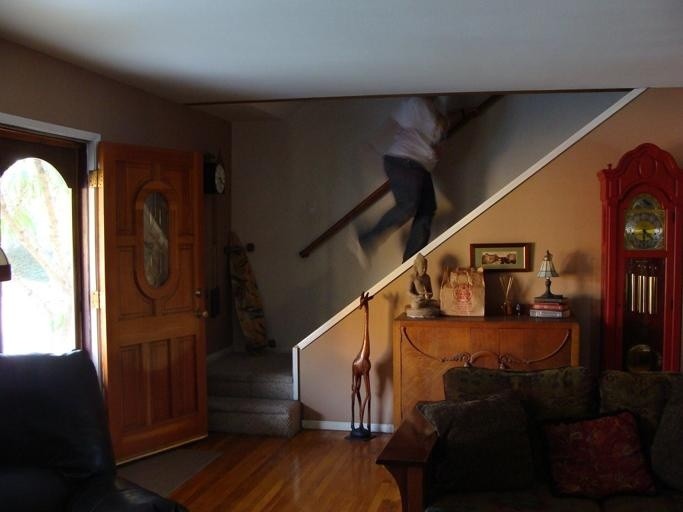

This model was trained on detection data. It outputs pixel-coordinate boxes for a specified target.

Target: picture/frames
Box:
[468,243,534,273]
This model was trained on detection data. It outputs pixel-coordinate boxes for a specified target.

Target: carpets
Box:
[115,438,221,498]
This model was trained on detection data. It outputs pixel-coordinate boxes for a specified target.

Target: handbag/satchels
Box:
[438,263,486,317]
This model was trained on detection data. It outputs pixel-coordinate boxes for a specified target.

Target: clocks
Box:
[596,149,683,373]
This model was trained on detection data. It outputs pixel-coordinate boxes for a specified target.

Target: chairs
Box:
[0,349,188,511]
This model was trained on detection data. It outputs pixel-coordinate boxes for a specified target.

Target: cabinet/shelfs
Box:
[392,311,581,430]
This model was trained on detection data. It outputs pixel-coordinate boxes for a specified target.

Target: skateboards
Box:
[223,232,275,355]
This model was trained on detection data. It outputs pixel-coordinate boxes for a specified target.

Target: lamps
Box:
[536,249,560,299]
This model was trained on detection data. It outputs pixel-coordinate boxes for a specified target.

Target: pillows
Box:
[415,388,659,498]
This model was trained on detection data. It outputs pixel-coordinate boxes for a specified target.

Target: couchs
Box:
[376,366,681,511]
